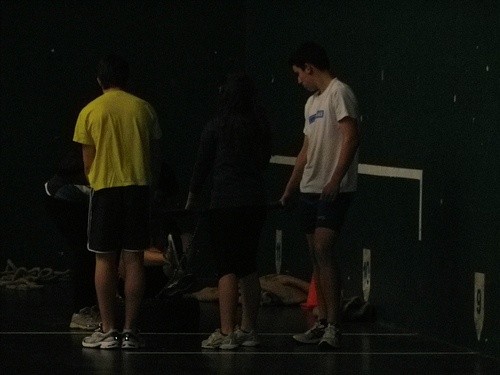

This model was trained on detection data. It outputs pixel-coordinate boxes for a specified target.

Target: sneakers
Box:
[69,306,101,330]
[121,327,146,349]
[318,323,341,349]
[81,322,121,348]
[91,305,102,321]
[233,324,261,346]
[292,315,328,343]
[82,348,116,373]
[201,327,241,349]
[203,349,233,368]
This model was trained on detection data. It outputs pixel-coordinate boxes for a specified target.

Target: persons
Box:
[81,51,163,350]
[42,165,188,330]
[278,42,360,348]
[184,73,273,350]
